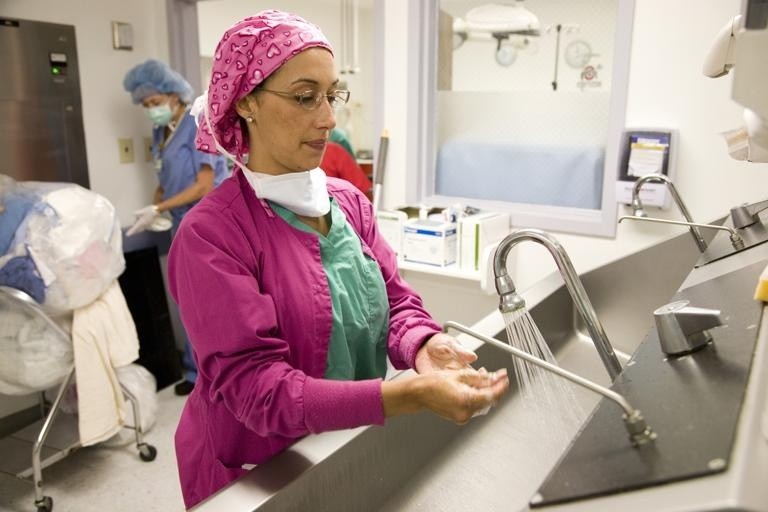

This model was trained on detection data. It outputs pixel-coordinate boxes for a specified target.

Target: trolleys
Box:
[0,284,157,511]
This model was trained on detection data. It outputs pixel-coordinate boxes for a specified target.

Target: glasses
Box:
[255,88,351,112]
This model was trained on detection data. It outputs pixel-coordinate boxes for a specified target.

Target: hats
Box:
[123,59,195,103]
[194,9,335,155]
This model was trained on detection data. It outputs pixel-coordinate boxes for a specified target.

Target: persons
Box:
[166,9,508,511]
[123,59,229,396]
[318,128,373,203]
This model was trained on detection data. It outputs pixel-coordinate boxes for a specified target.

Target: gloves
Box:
[125,205,173,238]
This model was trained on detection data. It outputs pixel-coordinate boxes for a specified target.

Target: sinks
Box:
[184,199,768,512]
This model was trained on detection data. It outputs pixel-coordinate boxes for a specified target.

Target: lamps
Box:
[452,3,540,68]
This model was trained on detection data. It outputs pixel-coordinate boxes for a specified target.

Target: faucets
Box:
[630,172,707,254]
[491,230,626,383]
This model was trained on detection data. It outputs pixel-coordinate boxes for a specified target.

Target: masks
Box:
[139,97,181,127]
[242,167,331,218]
